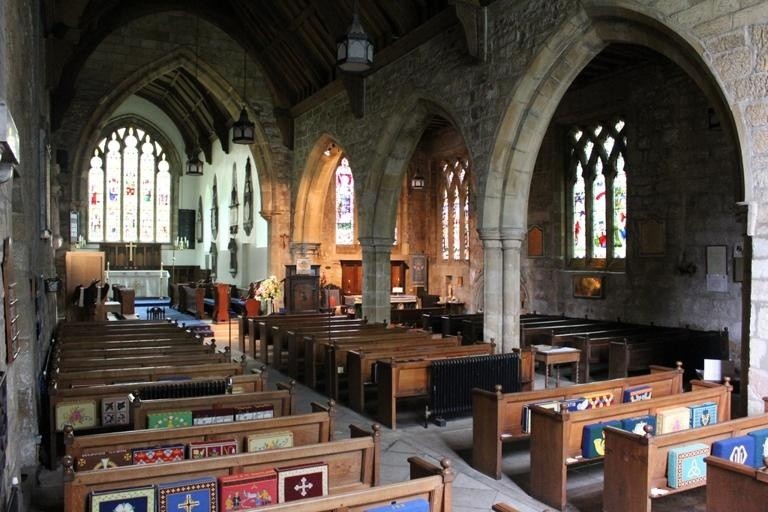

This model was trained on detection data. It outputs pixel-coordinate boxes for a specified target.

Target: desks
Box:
[103,269,169,301]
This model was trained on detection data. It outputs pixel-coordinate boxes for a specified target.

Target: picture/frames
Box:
[704,244,728,276]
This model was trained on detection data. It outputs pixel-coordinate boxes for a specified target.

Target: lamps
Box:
[411,145,426,191]
[185,12,205,177]
[231,44,258,144]
[334,0,377,72]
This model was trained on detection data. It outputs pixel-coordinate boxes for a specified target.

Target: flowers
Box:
[256,276,282,299]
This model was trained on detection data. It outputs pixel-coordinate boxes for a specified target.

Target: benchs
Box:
[43,296,767,511]
